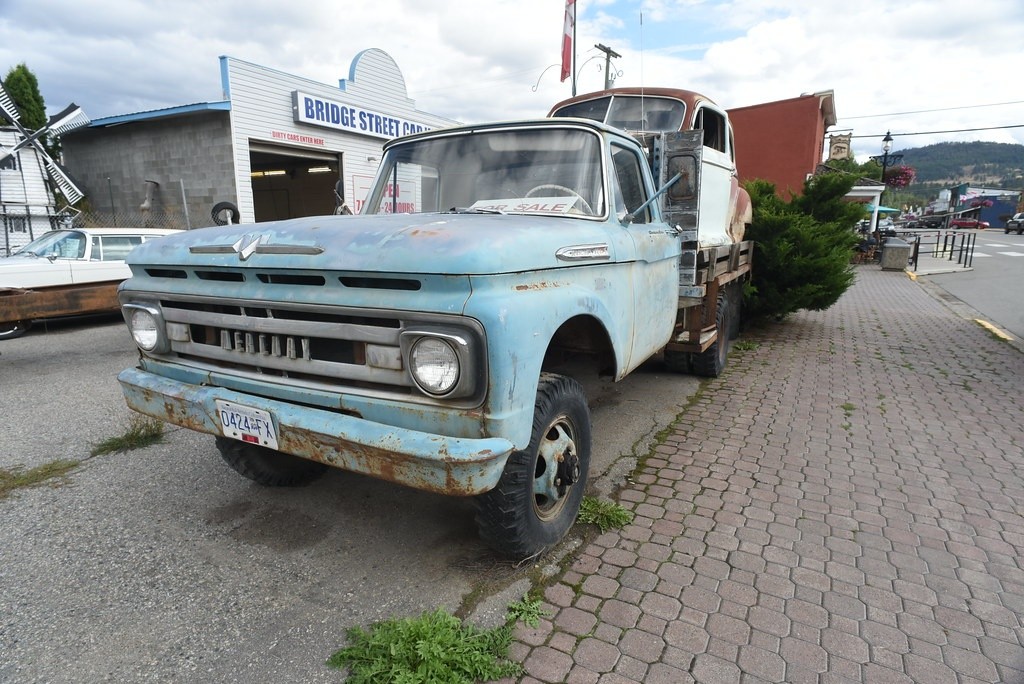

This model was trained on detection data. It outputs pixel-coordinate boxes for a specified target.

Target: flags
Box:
[560,0,575,83]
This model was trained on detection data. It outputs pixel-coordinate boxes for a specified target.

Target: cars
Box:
[906,221,938,229]
[1004,213,1024,235]
[854,219,896,237]
[948,217,989,229]
[0,228,188,340]
[544,87,752,247]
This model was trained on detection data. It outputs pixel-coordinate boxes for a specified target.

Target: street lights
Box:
[976,190,986,229]
[869,129,904,230]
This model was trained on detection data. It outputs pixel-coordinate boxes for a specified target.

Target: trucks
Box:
[117,117,756,563]
